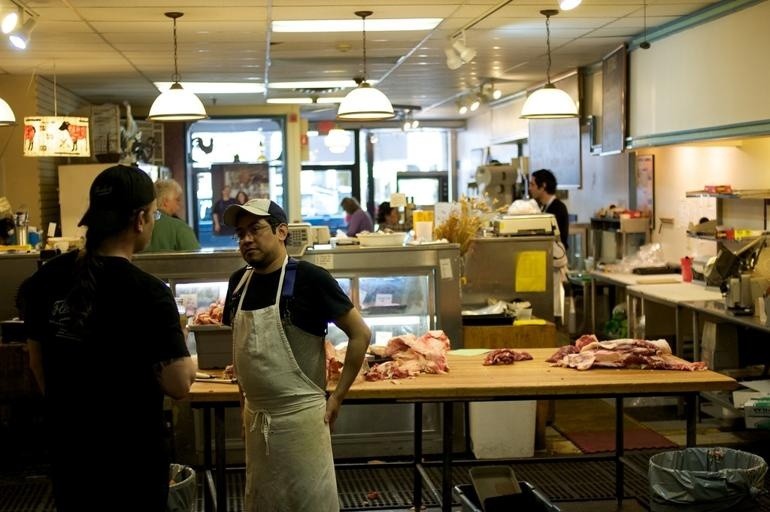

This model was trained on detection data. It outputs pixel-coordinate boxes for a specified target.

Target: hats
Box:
[78,164,155,227]
[223,199,287,227]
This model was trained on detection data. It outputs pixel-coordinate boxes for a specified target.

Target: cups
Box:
[414,210,436,240]
[680,258,693,282]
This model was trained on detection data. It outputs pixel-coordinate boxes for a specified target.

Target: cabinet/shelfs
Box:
[684,183,769,247]
[133,243,468,460]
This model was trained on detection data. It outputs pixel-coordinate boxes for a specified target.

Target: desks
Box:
[185,333,739,509]
[588,265,770,429]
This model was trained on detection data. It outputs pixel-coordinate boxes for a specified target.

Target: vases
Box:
[458,255,467,305]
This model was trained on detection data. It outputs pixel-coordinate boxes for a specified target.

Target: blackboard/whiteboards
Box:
[600,43,626,156]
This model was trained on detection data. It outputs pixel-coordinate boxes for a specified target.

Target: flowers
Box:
[431,187,509,254]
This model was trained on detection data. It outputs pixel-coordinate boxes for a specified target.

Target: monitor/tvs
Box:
[396,171,449,210]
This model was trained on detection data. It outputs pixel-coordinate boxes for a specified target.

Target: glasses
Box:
[232,223,280,242]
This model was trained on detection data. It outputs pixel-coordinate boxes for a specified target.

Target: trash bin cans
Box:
[648,446,768,511]
[168,463,196,511]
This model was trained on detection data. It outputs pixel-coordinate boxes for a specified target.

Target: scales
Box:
[490,213,561,236]
[286,223,330,257]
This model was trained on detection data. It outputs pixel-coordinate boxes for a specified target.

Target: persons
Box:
[528,170,569,330]
[221,198,372,512]
[235,191,249,204]
[213,186,236,235]
[377,200,413,231]
[148,177,202,250]
[342,199,373,238]
[15,164,198,511]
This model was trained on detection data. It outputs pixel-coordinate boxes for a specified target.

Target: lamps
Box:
[0,1,39,53]
[398,105,421,132]
[336,9,396,123]
[455,84,502,116]
[146,11,208,126]
[518,8,580,119]
[442,35,477,70]
